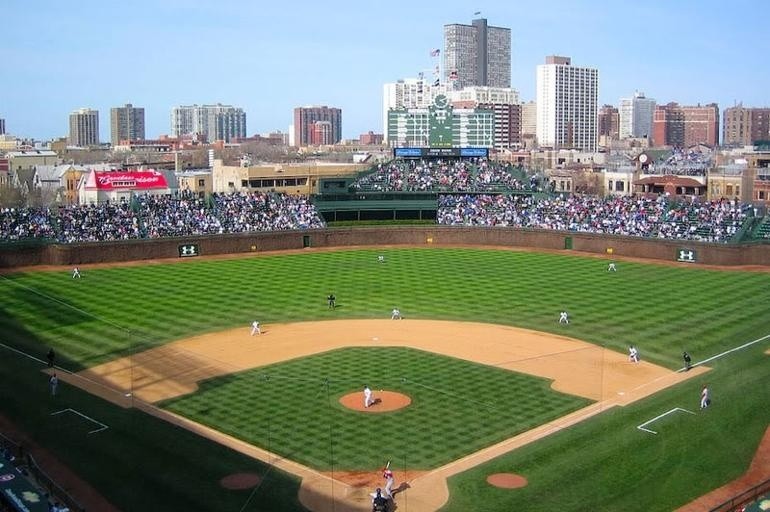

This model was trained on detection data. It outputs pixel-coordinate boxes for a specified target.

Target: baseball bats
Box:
[385,459,391,470]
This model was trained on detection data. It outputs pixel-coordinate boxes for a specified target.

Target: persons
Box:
[628,345,638,363]
[251,319,260,337]
[50,371,58,396]
[391,307,401,320]
[372,487,388,511]
[699,385,708,408]
[644,147,712,175]
[46,347,56,368]
[350,158,550,192]
[558,310,569,324]
[0,443,70,512]
[681,351,691,372]
[1,187,327,244]
[383,466,394,497]
[327,294,335,311]
[607,263,616,273]
[363,384,375,407]
[437,194,757,247]
[377,255,384,263]
[72,265,81,279]
